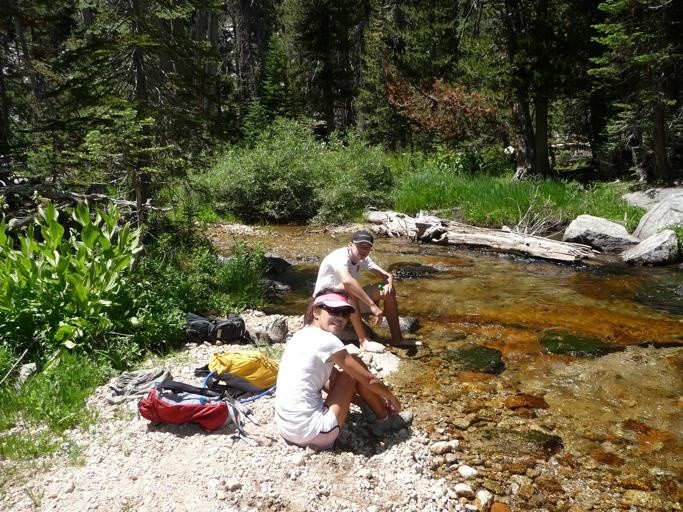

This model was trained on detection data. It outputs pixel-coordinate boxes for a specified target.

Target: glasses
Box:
[321,304,351,316]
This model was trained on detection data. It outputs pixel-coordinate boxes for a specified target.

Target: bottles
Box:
[378,284,384,293]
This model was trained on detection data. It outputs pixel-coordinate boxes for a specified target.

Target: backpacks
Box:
[106,351,279,432]
[186,312,289,345]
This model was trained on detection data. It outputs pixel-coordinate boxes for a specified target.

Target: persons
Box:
[274,287,413,450]
[312,232,423,353]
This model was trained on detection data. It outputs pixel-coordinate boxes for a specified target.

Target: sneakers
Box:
[367,412,413,435]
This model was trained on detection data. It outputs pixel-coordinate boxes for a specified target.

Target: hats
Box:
[351,230,374,244]
[312,293,356,308]
